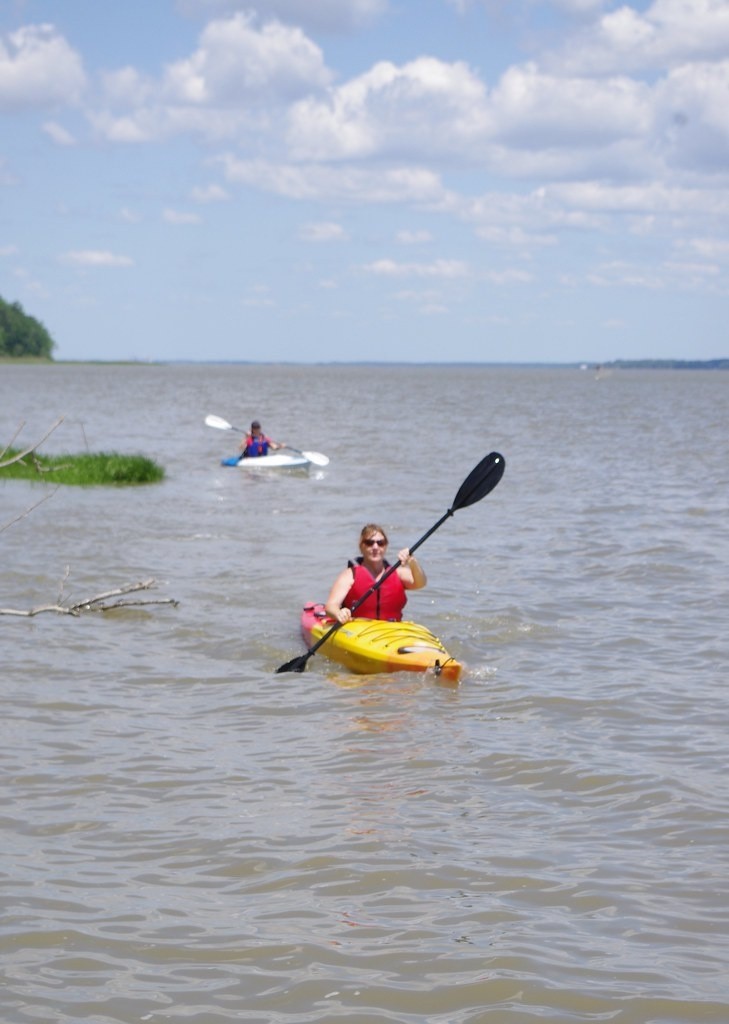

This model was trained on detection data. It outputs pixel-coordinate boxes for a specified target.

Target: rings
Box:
[344,615,347,617]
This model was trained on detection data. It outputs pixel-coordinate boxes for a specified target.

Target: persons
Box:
[239,422,286,456]
[325,524,427,625]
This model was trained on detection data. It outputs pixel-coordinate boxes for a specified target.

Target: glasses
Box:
[362,539,387,547]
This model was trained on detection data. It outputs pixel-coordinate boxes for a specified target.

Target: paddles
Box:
[206,412,330,468]
[273,451,507,678]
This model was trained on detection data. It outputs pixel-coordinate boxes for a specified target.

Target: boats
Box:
[219,455,312,477]
[301,601,462,689]
